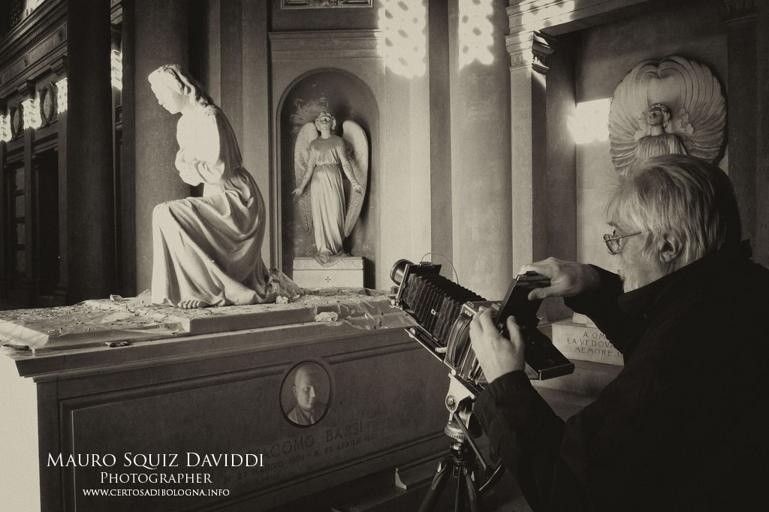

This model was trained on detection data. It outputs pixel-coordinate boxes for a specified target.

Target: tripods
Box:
[418,449,483,512]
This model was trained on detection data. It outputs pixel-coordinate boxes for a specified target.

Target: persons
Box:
[468,154,768,511]
[634,104,688,160]
[291,112,365,264]
[148,62,276,310]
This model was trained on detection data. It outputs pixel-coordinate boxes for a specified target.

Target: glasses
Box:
[602,229,643,254]
